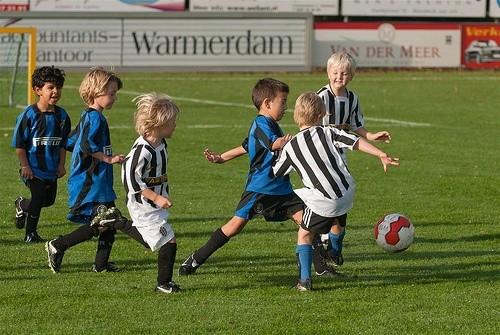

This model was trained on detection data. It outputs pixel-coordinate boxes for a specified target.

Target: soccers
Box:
[373,213,416,253]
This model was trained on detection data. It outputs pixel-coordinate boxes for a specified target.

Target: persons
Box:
[271,92,400,291]
[90,92,186,294]
[316,52,391,247]
[45,68,125,273]
[14,65,71,243]
[178,78,345,278]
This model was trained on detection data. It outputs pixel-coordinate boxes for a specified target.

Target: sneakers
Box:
[178,250,204,276]
[315,266,348,278]
[289,278,313,292]
[89,206,122,227]
[45,239,65,274]
[153,281,186,294]
[321,250,344,265]
[24,229,47,243]
[15,197,27,229]
[91,262,122,272]
